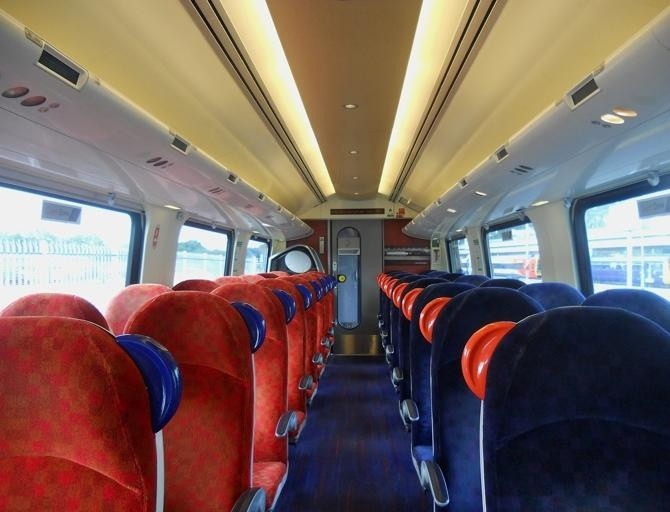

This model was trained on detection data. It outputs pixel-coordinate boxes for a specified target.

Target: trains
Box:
[459,229,670,302]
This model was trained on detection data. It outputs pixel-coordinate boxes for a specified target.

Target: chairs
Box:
[0,271,339,512]
[375,267,669,512]
